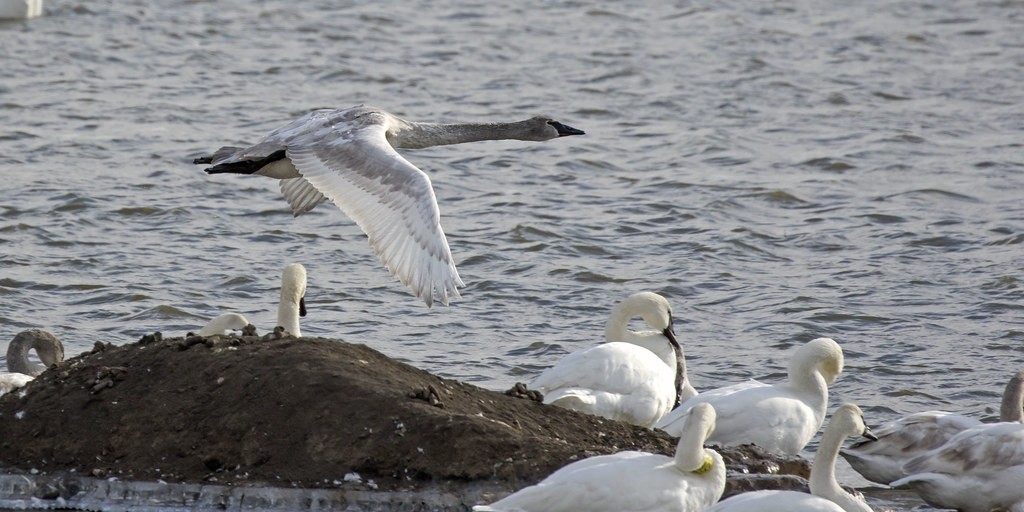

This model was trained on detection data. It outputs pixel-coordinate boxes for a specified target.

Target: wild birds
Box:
[474,291,1024,512]
[0,329,65,395]
[196,263,307,337]
[191,104,586,309]
